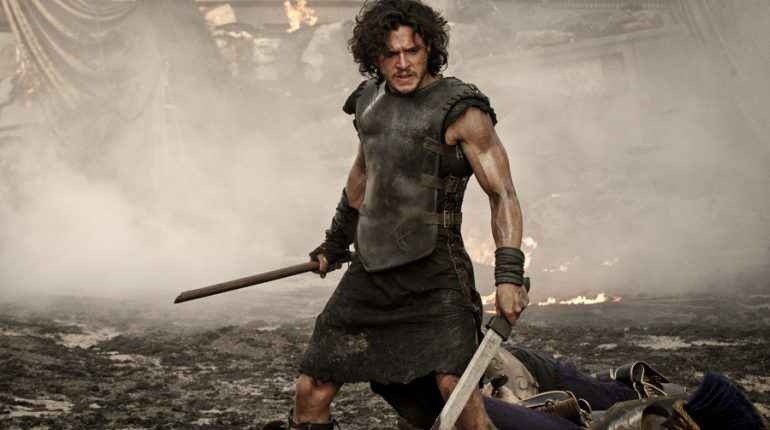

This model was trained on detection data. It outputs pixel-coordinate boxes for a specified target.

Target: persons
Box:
[287,0,529,430]
